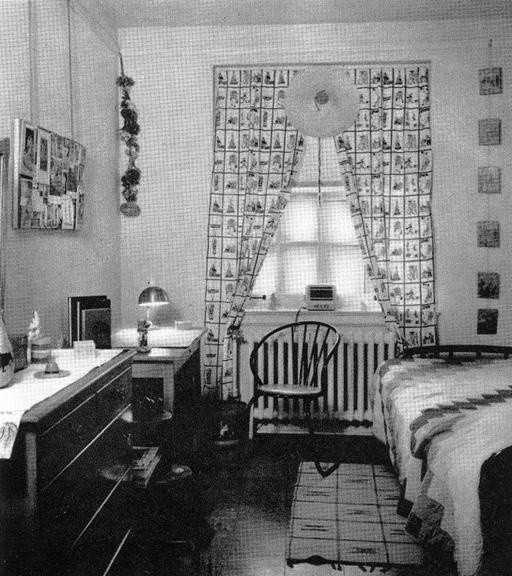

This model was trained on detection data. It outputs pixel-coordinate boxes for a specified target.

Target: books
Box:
[133,444,162,489]
[67,295,111,349]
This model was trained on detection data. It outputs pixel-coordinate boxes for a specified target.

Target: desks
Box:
[108,327,210,420]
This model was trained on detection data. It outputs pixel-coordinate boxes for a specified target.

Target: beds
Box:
[380,345,510,576]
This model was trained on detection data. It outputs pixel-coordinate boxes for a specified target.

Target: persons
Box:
[32,184,49,230]
[24,134,34,162]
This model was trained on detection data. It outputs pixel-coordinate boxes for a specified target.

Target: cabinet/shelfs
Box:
[1,348,142,576]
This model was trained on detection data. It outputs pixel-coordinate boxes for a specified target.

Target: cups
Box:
[31,344,52,364]
[7,334,27,373]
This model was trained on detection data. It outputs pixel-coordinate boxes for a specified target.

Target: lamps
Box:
[137,286,169,353]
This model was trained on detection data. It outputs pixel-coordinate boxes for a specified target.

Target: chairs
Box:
[241,321,344,477]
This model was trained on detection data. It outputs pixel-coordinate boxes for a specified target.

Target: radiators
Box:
[240,316,401,437]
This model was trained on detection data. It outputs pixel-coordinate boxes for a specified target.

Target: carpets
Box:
[284,460,424,568]
